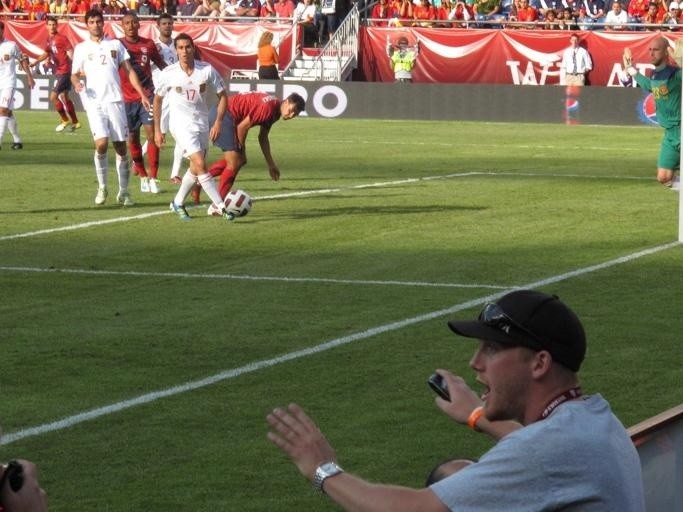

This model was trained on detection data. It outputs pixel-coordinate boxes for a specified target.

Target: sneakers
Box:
[221,208,232,221]
[114,192,133,206]
[169,176,185,184]
[192,179,200,205]
[94,190,107,205]
[139,176,149,193]
[206,205,223,217]
[68,123,80,131]
[149,179,159,196]
[55,120,69,133]
[10,142,23,151]
[169,202,189,220]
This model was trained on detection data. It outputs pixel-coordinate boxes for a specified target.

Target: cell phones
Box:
[428,372,451,402]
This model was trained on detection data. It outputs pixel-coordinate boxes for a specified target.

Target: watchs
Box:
[315,462,343,494]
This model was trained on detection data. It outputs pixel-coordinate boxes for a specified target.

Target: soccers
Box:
[224,190,252,217]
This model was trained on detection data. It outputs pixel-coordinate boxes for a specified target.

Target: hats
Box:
[667,2,683,12]
[446,290,586,372]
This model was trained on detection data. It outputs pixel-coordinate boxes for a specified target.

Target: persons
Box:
[238,0,262,17]
[519,0,540,29]
[181,0,198,21]
[2,0,12,20]
[62,0,73,14]
[30,18,83,134]
[274,0,297,24]
[628,0,650,31]
[394,0,414,27]
[646,2,665,32]
[622,37,681,184]
[116,13,169,193]
[294,0,317,46]
[561,34,594,85]
[72,11,151,207]
[541,0,558,11]
[220,0,238,23]
[260,1,275,17]
[140,1,156,15]
[544,11,561,30]
[386,35,420,82]
[127,0,140,14]
[91,0,109,14]
[317,0,335,48]
[662,1,683,32]
[560,8,581,30]
[104,1,124,14]
[68,1,90,14]
[133,15,183,184]
[371,0,390,28]
[509,0,522,28]
[560,0,578,9]
[32,0,50,20]
[580,1,607,30]
[606,1,627,30]
[454,0,474,28]
[413,0,436,27]
[1,459,49,511]
[0,21,36,150]
[266,292,645,510]
[11,0,34,20]
[50,1,66,19]
[193,93,307,216]
[158,0,178,21]
[257,32,282,81]
[435,0,455,26]
[154,35,235,221]
[194,1,222,23]
[472,0,503,29]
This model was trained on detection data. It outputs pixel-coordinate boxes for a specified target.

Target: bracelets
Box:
[466,406,485,429]
